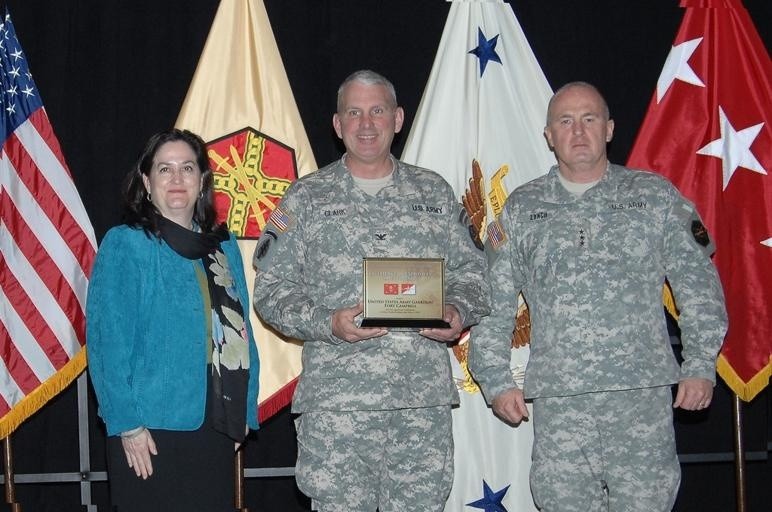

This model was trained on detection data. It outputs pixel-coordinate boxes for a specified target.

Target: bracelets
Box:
[119,426,146,439]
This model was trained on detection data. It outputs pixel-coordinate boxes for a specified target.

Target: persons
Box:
[252,68,492,512]
[466,80,730,512]
[86,125,261,512]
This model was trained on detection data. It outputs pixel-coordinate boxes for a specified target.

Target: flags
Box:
[628,1,772,404]
[399,0,557,511]
[0,1,102,443]
[174,0,320,453]
